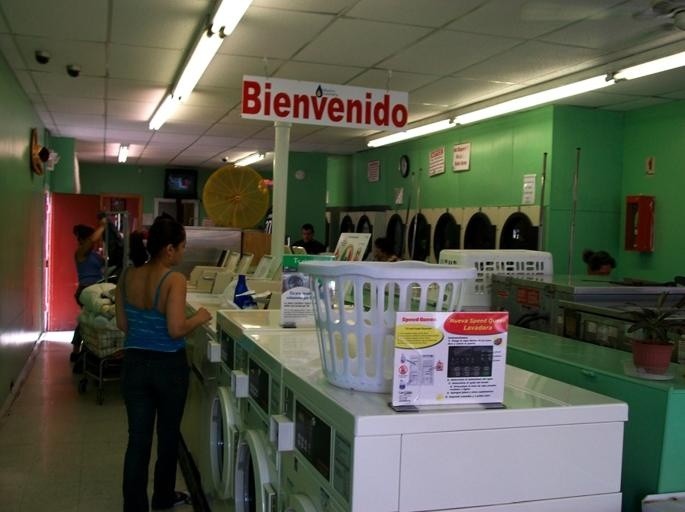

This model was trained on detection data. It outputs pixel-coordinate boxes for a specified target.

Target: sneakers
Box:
[174,490,191,508]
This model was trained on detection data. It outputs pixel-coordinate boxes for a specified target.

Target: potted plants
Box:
[625,290,678,374]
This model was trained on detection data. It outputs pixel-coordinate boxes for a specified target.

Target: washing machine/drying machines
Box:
[281,349,629,511]
[228,327,396,511]
[199,308,368,511]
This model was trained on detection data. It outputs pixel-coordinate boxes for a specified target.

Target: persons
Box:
[291,224,326,254]
[115,213,213,512]
[583,250,616,275]
[375,236,403,262]
[70,217,113,374]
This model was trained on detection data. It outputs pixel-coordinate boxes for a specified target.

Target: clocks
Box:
[400,156,408,177]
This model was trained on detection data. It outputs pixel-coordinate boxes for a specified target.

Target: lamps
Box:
[366,113,456,149]
[617,51,685,82]
[235,151,265,170]
[171,25,236,101]
[118,143,129,163]
[209,0,253,38]
[148,95,182,131]
[456,73,617,128]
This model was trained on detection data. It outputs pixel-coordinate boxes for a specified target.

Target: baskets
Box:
[437,249,554,308]
[299,260,478,395]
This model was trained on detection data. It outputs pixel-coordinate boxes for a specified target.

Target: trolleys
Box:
[76,350,126,406]
